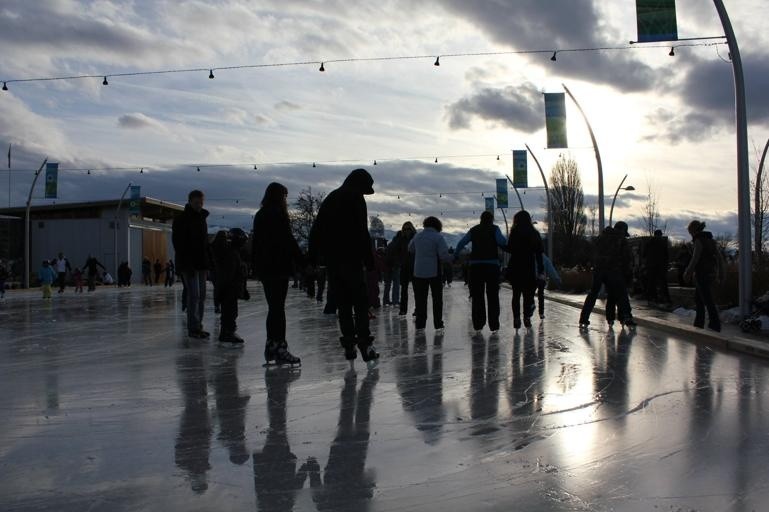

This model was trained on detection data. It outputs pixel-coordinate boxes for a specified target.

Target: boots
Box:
[265,339,278,359]
[272,340,300,363]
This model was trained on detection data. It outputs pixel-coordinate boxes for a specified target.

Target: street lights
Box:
[609,174,636,228]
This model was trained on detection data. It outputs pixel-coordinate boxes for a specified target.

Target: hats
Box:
[614,221,630,237]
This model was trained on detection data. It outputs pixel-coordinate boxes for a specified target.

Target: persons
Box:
[102,272,113,285]
[142,256,175,288]
[41,253,107,302]
[452,210,562,335]
[172,190,250,349]
[306,168,382,370]
[292,216,454,331]
[117,261,132,288]
[578,222,725,332]
[557,263,585,272]
[249,181,303,369]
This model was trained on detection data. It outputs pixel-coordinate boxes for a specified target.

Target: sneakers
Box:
[345,344,358,359]
[357,345,380,360]
